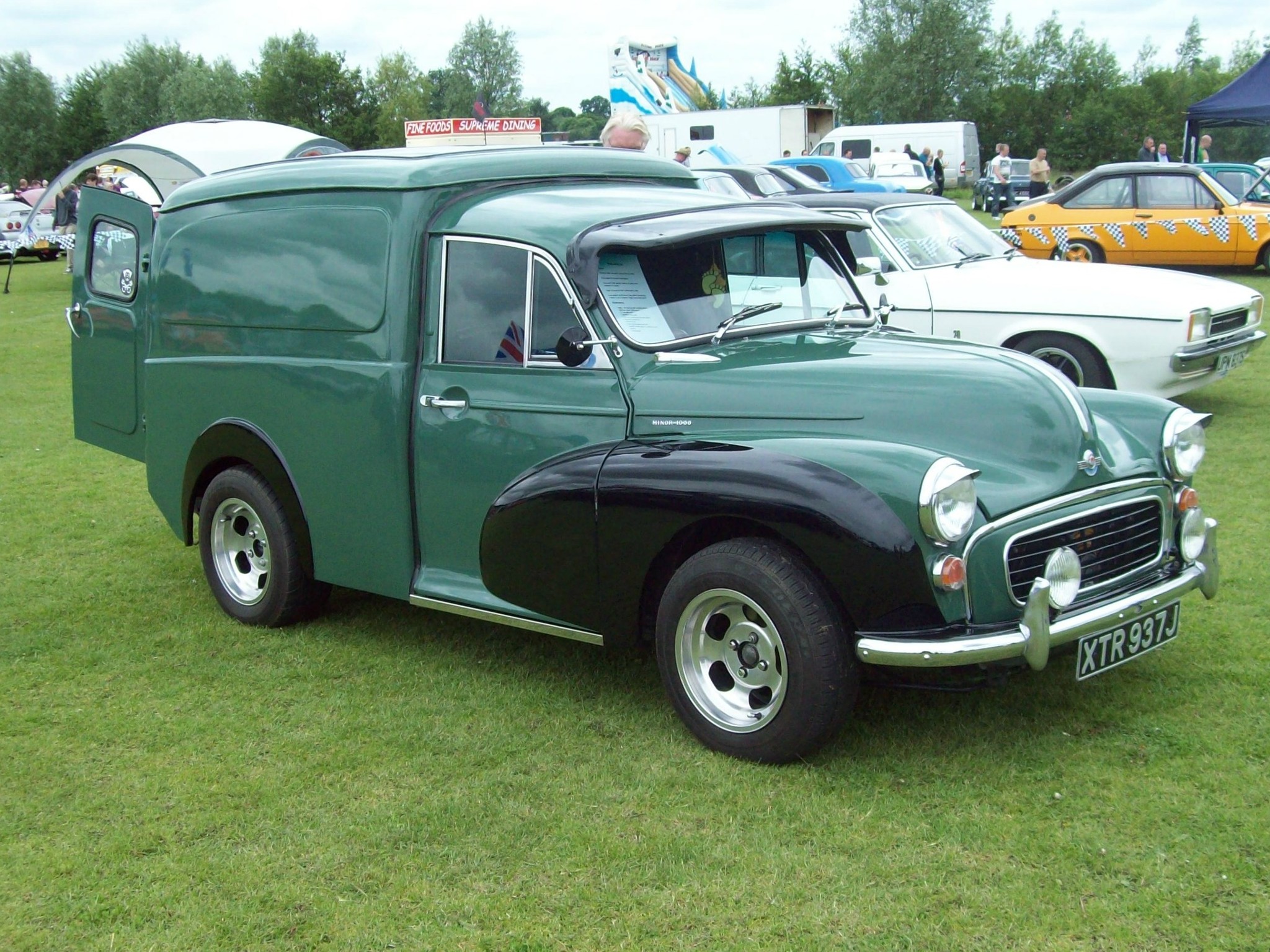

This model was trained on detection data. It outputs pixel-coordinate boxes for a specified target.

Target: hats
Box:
[674,148,690,156]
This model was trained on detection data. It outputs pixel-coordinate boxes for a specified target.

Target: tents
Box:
[1182,49,1270,164]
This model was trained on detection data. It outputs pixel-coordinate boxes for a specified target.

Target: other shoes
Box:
[66,268,74,273]
[991,216,1001,220]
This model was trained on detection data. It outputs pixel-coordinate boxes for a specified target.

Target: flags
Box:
[494,321,524,363]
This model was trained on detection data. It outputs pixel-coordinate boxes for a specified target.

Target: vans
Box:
[808,120,981,189]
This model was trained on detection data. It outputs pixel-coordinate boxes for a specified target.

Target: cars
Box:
[971,159,1052,213]
[0,172,138,261]
[64,141,1221,766]
[687,153,1270,400]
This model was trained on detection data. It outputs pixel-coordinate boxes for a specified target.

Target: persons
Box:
[991,143,1014,220]
[784,150,791,157]
[1154,142,1172,162]
[601,111,651,151]
[842,149,853,159]
[673,146,691,163]
[660,71,664,78]
[933,149,945,196]
[1197,135,1212,163]
[903,143,919,160]
[98,176,120,192]
[917,146,934,181]
[52,185,78,274]
[874,146,880,152]
[1139,136,1155,162]
[85,173,99,187]
[1030,148,1054,199]
[15,179,48,203]
[0,183,9,194]
[802,150,808,156]
